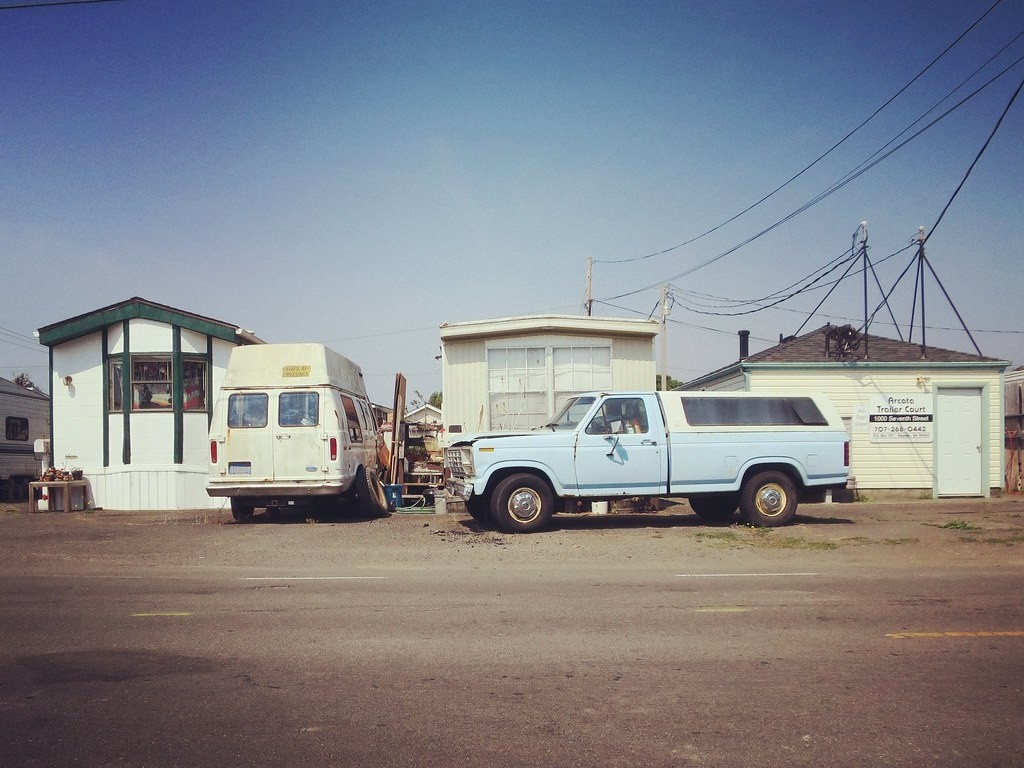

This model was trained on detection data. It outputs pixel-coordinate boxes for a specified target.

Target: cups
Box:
[72,470,83,480]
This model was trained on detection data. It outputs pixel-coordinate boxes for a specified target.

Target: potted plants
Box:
[44,466,83,481]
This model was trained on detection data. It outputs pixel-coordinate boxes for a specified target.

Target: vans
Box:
[205,344,383,508]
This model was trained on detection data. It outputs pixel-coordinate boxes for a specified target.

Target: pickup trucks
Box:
[436,386,857,534]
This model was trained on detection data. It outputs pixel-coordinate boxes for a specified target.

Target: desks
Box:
[28,480,87,513]
[410,472,442,483]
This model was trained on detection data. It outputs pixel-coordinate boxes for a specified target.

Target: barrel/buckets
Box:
[386,484,402,508]
[592,502,607,514]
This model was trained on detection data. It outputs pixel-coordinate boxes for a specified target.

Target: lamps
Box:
[63,376,73,386]
[916,374,926,384]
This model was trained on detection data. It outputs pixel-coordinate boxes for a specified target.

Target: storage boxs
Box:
[353,484,402,508]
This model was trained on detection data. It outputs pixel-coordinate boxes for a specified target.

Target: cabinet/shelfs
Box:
[404,422,445,482]
